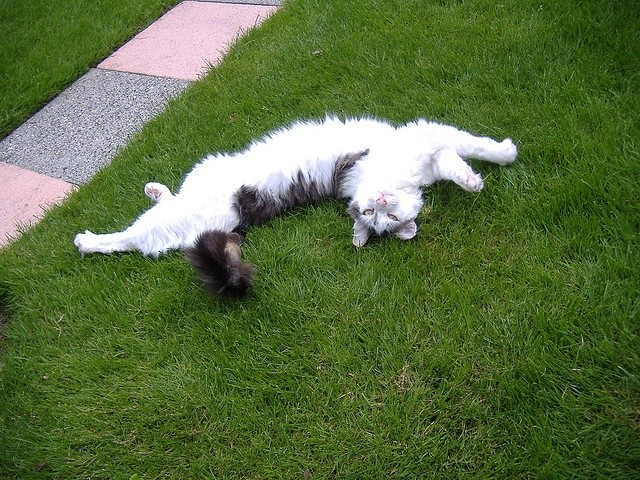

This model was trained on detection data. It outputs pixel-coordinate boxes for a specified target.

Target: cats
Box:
[74,113,517,295]
